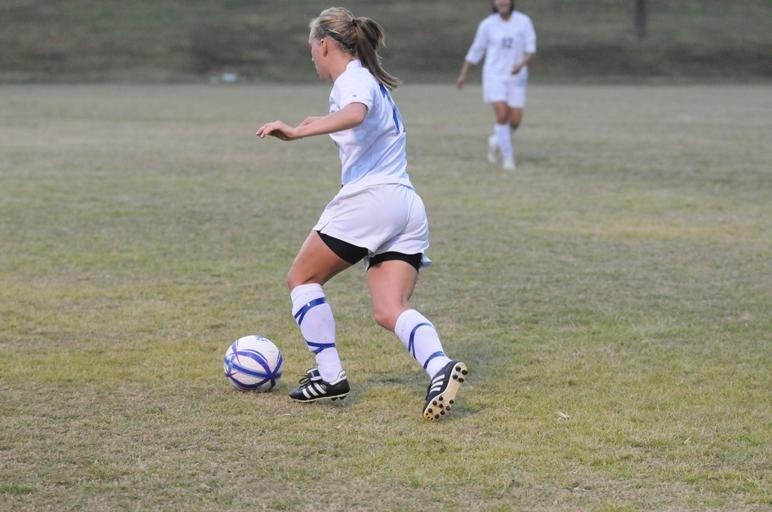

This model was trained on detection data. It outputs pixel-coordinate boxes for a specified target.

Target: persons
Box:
[454,1,537,175]
[254,7,470,422]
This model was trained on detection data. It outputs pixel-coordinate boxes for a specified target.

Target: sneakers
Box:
[288,370,351,403]
[419,359,468,423]
[486,135,501,164]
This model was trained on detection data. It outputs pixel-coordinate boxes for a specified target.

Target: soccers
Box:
[224,335,282,392]
